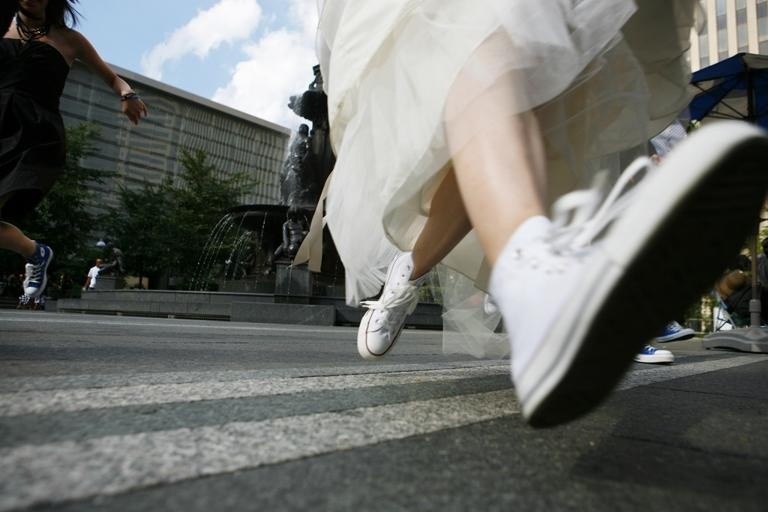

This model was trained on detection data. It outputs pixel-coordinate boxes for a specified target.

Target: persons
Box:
[81,258,104,291]
[282,205,309,260]
[0,0,148,298]
[355,0,768,431]
[280,123,311,205]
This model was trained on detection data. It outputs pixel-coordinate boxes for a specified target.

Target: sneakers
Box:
[490,120,768,428]
[633,345,675,364]
[655,322,696,342]
[23,242,54,297]
[357,253,428,359]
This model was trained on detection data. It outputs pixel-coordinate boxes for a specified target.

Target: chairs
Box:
[709,286,752,331]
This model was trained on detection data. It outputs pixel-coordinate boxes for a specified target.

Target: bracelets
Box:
[120,90,143,103]
